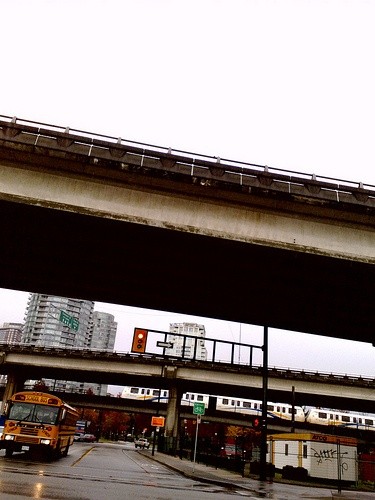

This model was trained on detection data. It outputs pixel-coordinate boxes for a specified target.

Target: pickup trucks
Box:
[134,438,150,449]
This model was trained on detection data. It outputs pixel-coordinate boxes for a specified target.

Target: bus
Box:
[0,391,79,463]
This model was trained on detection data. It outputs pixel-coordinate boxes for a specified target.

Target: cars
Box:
[73,433,97,444]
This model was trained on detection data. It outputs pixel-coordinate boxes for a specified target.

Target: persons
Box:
[94,429,101,442]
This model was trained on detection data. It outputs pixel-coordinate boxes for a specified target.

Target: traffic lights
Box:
[252,416,264,430]
[207,396,217,415]
[131,327,148,353]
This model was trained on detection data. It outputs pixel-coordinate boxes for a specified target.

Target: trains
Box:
[105,384,375,431]
